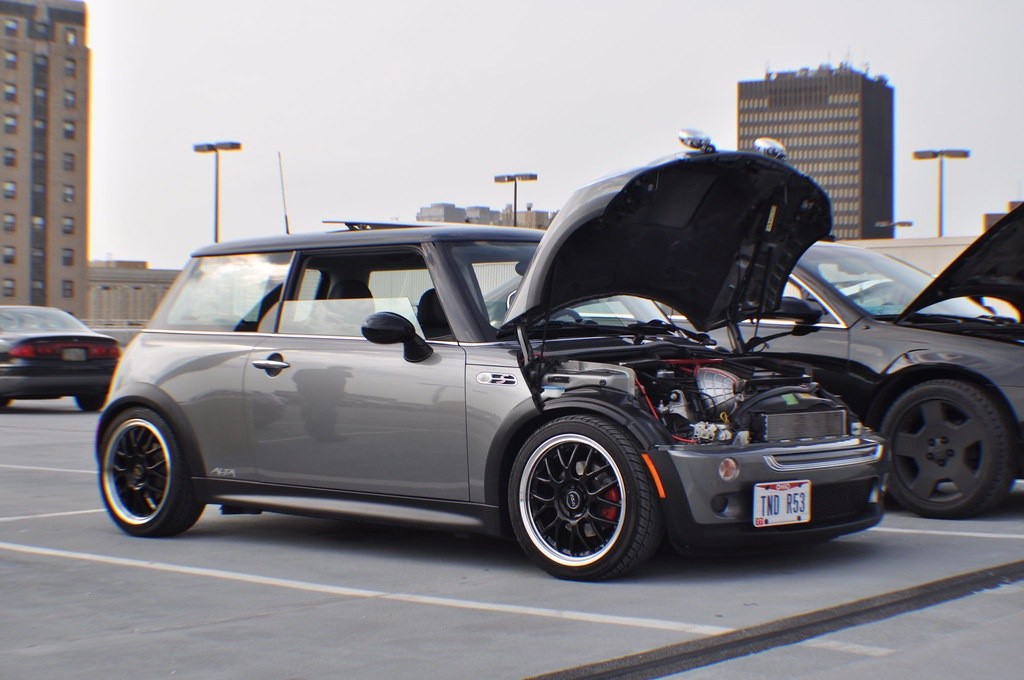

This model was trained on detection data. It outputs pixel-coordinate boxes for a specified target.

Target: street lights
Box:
[492,172,538,226]
[192,140,244,243]
[909,148,971,238]
[873,220,913,239]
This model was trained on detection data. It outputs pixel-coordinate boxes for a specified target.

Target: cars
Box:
[480,200,1024,522]
[93,125,894,584]
[0,305,123,414]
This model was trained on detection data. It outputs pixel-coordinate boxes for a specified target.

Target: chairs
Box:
[325,281,375,324]
[417,287,453,339]
